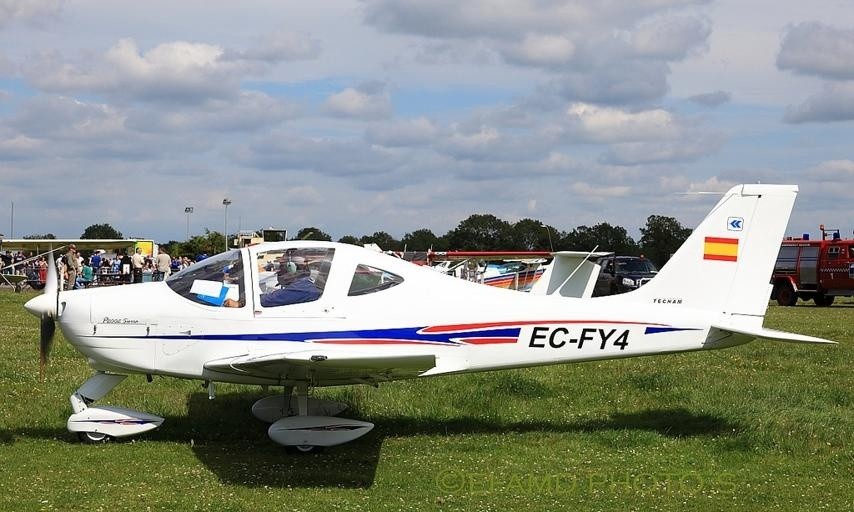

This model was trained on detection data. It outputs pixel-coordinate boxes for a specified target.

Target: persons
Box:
[0,244,210,290]
[223,248,321,308]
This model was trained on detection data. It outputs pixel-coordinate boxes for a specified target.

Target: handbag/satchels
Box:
[61,256,67,265]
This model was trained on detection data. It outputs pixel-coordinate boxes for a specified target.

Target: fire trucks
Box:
[770,224,854,306]
